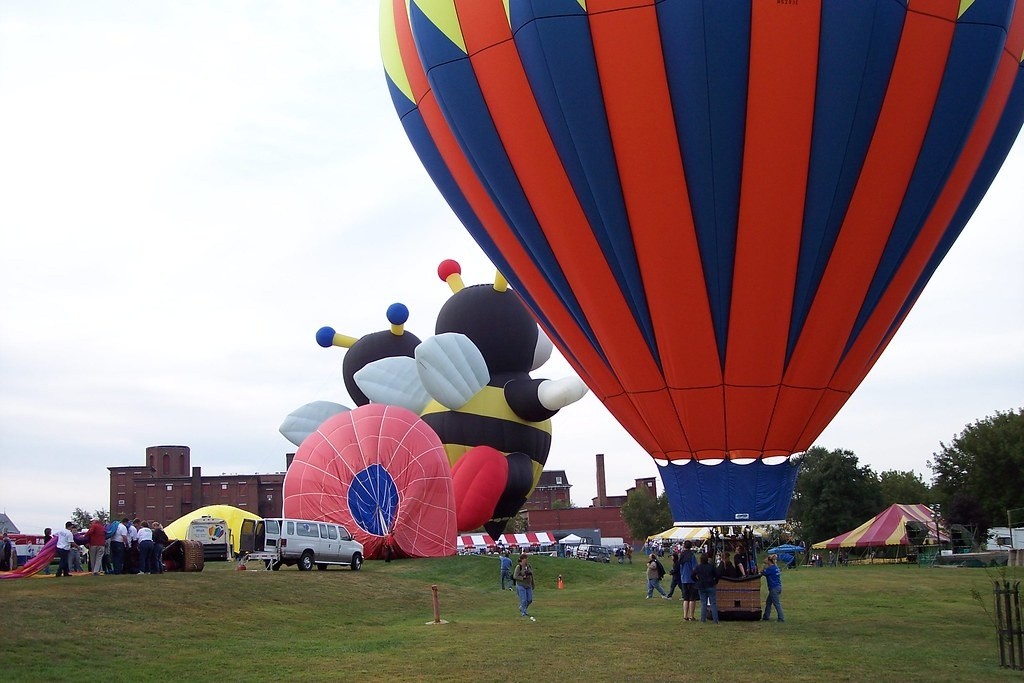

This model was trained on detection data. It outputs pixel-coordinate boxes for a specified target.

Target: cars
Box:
[585,545,610,562]
[576,544,590,559]
[614,547,626,557]
[239,518,364,572]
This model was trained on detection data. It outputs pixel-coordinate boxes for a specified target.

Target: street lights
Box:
[929,504,941,556]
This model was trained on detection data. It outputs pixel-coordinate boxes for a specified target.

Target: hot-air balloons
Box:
[0,520,204,579]
[378,0,1023,622]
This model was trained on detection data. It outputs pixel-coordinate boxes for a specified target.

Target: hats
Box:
[90,517,100,522]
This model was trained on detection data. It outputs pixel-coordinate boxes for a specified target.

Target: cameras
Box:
[524,570,532,578]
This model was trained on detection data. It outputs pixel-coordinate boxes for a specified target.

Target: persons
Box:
[498,554,513,591]
[811,551,848,567]
[43,518,169,577]
[646,540,748,624]
[0,533,14,572]
[513,555,535,616]
[627,546,632,563]
[613,546,626,564]
[761,554,784,622]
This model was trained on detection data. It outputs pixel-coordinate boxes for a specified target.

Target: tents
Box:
[812,503,951,565]
[457,531,556,555]
[768,544,804,571]
[559,534,586,556]
[640,525,794,552]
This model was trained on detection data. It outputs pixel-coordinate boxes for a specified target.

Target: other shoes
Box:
[509,588,513,592]
[679,598,684,601]
[689,617,696,621]
[777,619,784,622]
[763,618,770,620]
[56,574,61,577]
[64,574,73,577]
[683,617,689,621]
[93,573,98,576]
[646,596,653,599]
[662,596,665,599]
[145,572,150,574]
[665,597,672,600]
[138,571,144,575]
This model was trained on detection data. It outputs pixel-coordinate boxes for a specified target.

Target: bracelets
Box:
[763,561,766,564]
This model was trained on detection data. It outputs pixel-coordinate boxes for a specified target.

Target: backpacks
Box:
[510,564,522,586]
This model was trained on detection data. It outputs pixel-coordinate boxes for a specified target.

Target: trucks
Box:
[600,537,634,552]
[185,516,229,562]
[0,534,46,565]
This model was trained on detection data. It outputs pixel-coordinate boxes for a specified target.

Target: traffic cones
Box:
[558,574,564,589]
